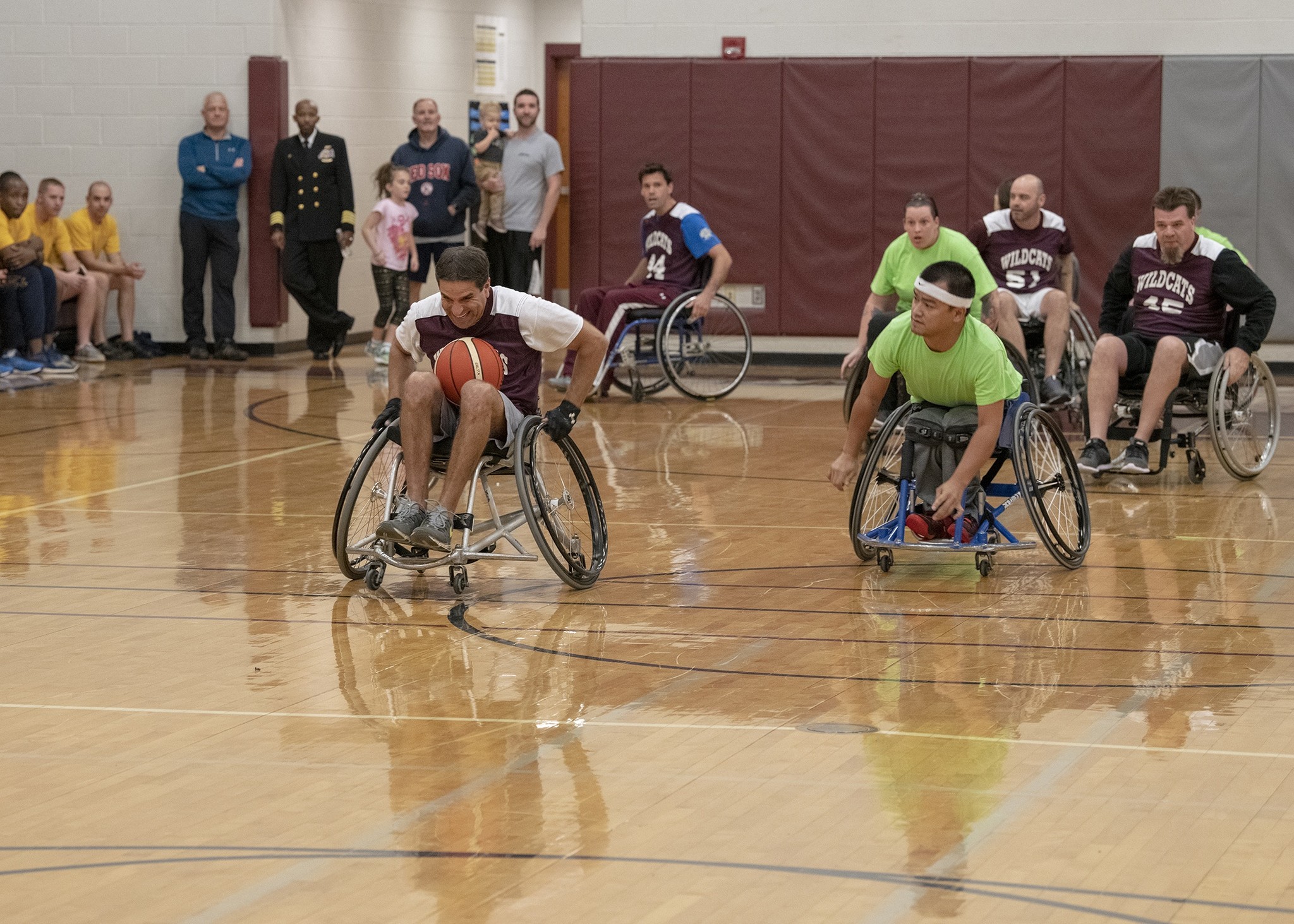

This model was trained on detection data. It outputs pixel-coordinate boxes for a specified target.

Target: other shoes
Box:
[186,363,238,385]
[28,343,81,373]
[332,314,353,358]
[365,366,389,390]
[364,340,390,364]
[187,337,209,359]
[1119,500,1150,526]
[472,222,488,242]
[75,363,106,383]
[873,412,886,426]
[312,351,329,360]
[96,340,134,360]
[487,219,508,233]
[0,375,43,392]
[215,338,248,361]
[5,355,43,374]
[332,360,346,388]
[546,376,571,391]
[1041,374,1072,404]
[73,344,106,362]
[121,338,154,358]
[0,364,14,379]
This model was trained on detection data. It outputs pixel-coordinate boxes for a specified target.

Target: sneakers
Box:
[947,515,978,543]
[375,494,428,543]
[1119,437,1151,473]
[906,511,952,540]
[410,498,461,552]
[1076,440,1113,474]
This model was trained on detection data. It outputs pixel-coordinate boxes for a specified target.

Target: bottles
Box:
[336,228,352,258]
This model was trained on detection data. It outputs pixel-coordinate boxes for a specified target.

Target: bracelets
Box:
[373,248,382,258]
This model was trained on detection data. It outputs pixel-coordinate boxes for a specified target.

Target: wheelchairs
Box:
[331,406,609,596]
[556,256,752,404]
[842,306,1101,577]
[1080,309,1282,484]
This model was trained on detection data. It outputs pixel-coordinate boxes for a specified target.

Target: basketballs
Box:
[433,337,503,408]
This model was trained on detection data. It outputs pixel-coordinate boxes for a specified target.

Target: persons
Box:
[389,97,481,305]
[17,177,106,365]
[176,90,252,361]
[962,172,1080,405]
[826,260,1023,543]
[0,171,81,379]
[64,181,155,360]
[361,161,422,366]
[1184,187,1258,312]
[545,161,734,396]
[839,192,1000,434]
[480,88,565,294]
[371,247,609,550]
[469,100,516,241]
[269,100,355,360]
[1076,189,1277,474]
